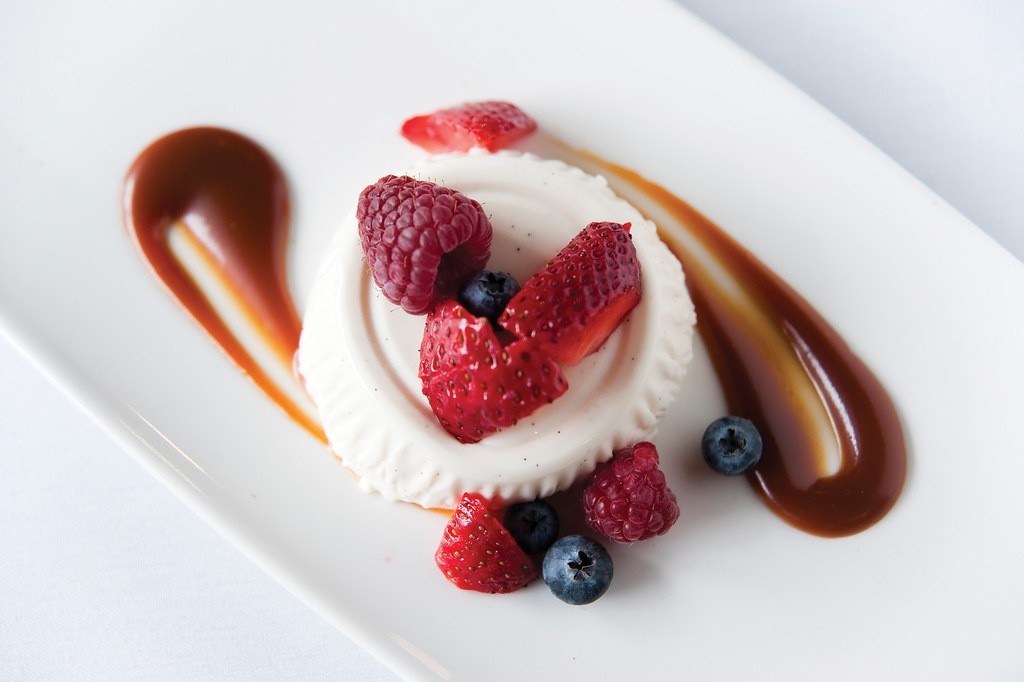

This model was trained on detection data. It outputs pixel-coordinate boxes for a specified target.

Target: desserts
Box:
[119,102,906,607]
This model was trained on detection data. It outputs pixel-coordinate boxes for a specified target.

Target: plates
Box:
[0,1,1023,682]
